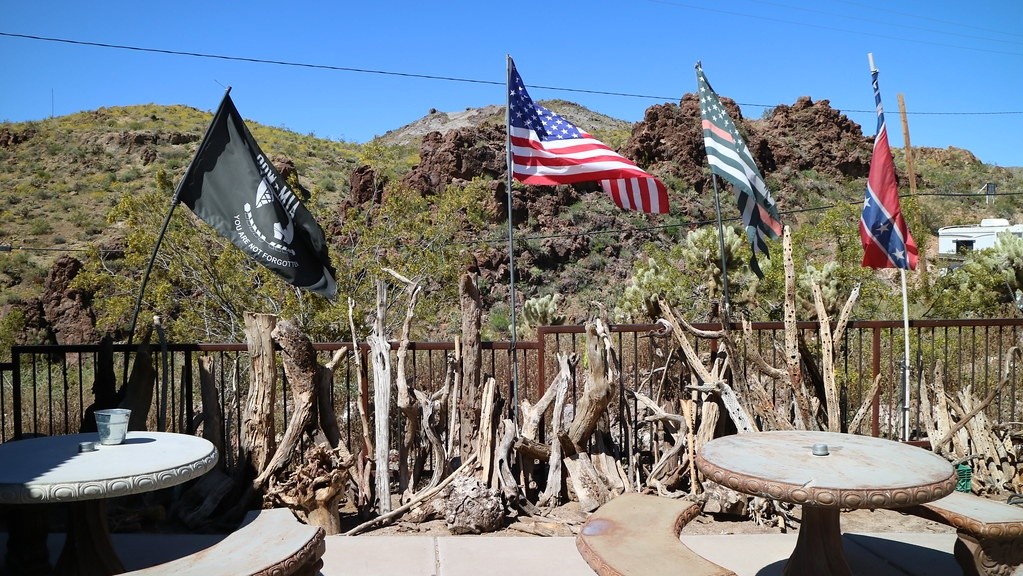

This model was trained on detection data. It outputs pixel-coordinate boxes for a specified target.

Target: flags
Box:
[695,66,783,284]
[859,73,919,271]
[175,94,337,300]
[508,56,670,215]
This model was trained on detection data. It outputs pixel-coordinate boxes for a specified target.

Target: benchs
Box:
[578,492,738,576]
[885,489,1023,576]
[115,507,322,576]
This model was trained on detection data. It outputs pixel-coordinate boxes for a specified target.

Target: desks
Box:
[699,430,956,576]
[1,430,218,576]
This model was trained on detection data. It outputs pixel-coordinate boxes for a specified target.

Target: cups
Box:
[93,408,132,445]
